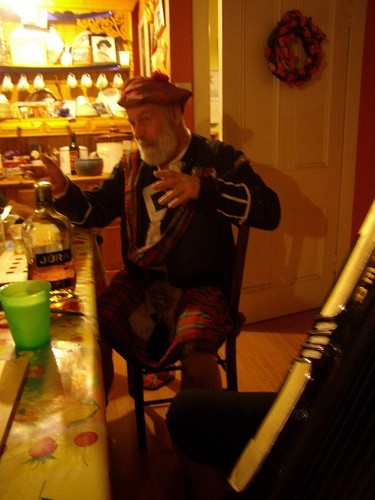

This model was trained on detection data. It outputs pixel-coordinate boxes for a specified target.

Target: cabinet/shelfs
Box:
[0,57,137,284]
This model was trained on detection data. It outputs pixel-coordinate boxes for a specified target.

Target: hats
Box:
[117,71,192,109]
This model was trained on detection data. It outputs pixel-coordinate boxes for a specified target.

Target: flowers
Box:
[261,10,330,89]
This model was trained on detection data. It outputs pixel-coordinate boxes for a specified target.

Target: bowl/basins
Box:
[74,159,103,176]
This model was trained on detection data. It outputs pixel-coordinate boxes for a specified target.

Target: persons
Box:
[20,72,282,392]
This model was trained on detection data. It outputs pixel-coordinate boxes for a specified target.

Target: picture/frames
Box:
[136,0,166,79]
[88,33,119,63]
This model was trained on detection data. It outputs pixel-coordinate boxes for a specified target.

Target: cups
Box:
[9,224,24,255]
[0,280,52,352]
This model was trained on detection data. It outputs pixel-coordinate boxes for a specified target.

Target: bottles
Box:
[21,181,76,309]
[69,133,80,175]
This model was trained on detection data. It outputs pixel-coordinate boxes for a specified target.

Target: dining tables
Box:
[0,225,114,500]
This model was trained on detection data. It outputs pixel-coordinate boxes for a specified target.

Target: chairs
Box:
[127,220,250,455]
